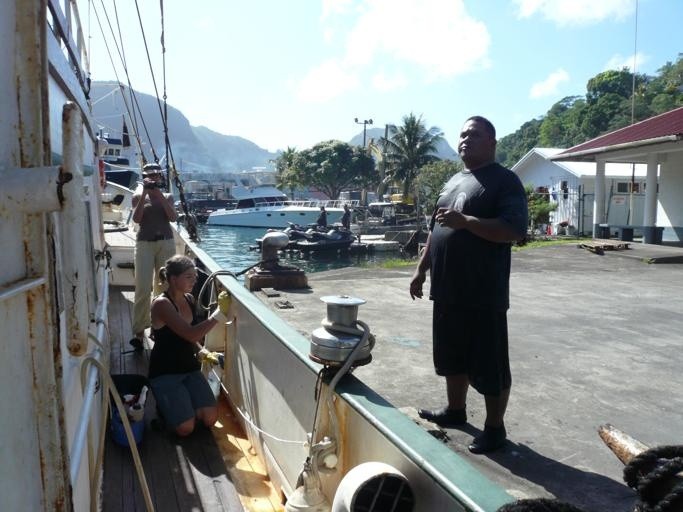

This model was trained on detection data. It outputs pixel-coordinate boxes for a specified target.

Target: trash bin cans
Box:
[601,227,609,238]
[654,227,663,243]
[621,227,633,241]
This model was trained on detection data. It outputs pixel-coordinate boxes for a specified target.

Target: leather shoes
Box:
[418,403,467,424]
[468,419,506,454]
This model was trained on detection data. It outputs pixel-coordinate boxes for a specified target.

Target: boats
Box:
[204,184,360,229]
[0,0,526,512]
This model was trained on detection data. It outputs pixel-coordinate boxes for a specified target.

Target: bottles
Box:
[128,403,144,422]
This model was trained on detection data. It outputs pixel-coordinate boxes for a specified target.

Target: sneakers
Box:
[130,338,144,352]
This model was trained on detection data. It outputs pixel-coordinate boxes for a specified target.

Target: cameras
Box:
[145,180,159,191]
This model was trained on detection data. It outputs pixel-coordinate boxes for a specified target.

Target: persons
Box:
[408,117,529,452]
[127,162,177,349]
[316,205,329,227]
[146,254,231,437]
[338,203,350,226]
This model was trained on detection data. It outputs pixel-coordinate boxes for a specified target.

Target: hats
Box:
[142,163,163,175]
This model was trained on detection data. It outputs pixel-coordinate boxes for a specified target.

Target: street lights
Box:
[353,116,373,147]
